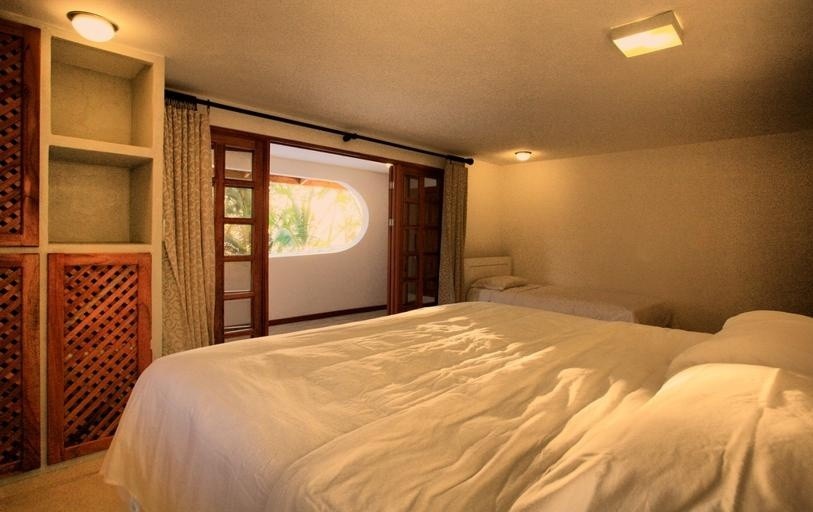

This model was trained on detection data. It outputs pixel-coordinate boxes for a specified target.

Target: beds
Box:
[107,298,810,512]
[459,255,670,327]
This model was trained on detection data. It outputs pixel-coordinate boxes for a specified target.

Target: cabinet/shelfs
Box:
[0,13,171,488]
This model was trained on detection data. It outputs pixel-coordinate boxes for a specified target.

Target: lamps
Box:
[61,8,122,48]
[514,150,532,162]
[607,11,689,61]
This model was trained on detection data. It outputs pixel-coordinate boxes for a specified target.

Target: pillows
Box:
[621,310,809,510]
[469,273,529,293]
